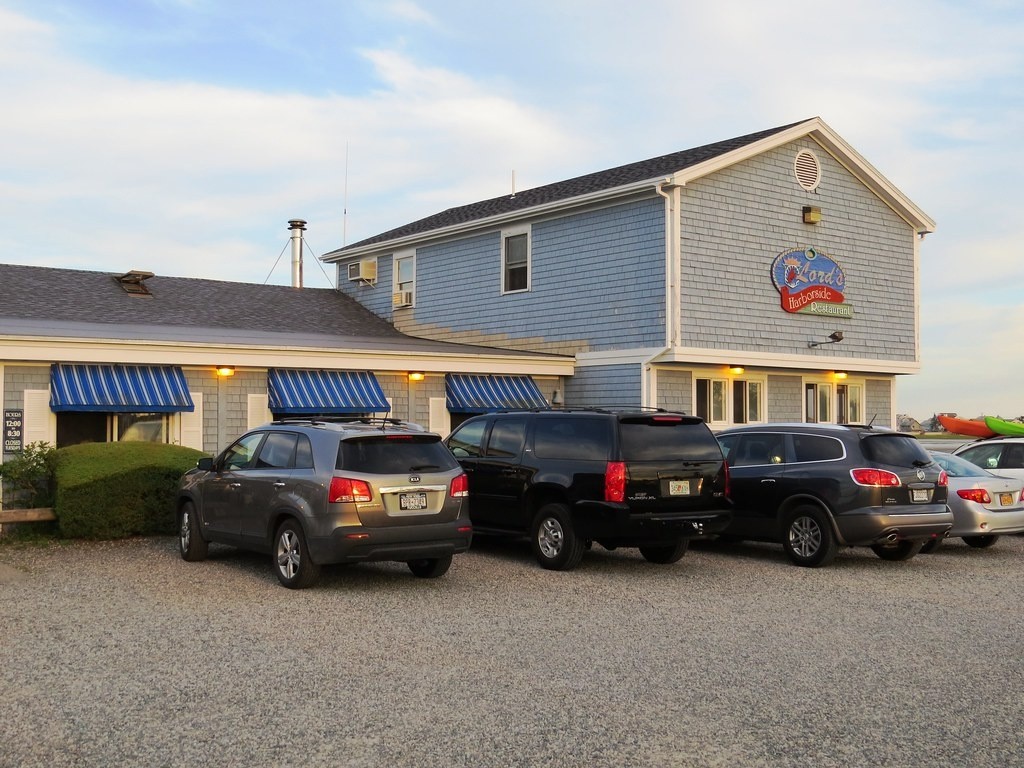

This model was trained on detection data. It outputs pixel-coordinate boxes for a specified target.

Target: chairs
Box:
[497,427,520,453]
[737,442,768,465]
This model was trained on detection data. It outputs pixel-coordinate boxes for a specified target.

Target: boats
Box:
[985,417,1024,436]
[937,415,998,439]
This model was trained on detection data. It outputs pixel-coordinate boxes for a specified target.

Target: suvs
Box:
[442,401,735,573]
[948,435,1024,485]
[172,415,473,591]
[704,422,955,570]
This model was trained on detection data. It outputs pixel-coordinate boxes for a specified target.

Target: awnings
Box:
[47,364,196,412]
[266,369,390,413]
[444,374,553,412]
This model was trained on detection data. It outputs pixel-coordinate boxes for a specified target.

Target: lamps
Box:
[834,370,847,378]
[552,389,564,404]
[730,365,744,374]
[808,331,843,348]
[408,371,425,381]
[216,365,235,376]
[802,205,820,223]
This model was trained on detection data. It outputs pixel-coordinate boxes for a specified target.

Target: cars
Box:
[928,451,1024,547]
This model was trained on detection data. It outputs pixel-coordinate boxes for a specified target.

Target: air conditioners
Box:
[393,291,413,307]
[348,261,376,282]
